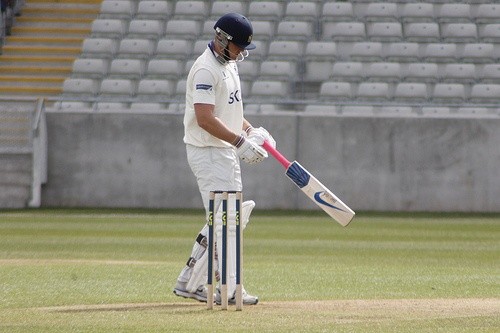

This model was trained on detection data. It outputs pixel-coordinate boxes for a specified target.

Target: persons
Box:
[173,13,276,305]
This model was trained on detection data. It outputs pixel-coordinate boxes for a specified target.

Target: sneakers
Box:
[216,285,258,305]
[173,280,208,302]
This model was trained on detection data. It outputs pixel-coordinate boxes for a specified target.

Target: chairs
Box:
[54,0,500,113]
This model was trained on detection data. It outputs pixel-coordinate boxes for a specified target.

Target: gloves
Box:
[235,139,268,164]
[248,127,276,151]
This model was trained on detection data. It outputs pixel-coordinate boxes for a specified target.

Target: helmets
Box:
[213,13,256,49]
